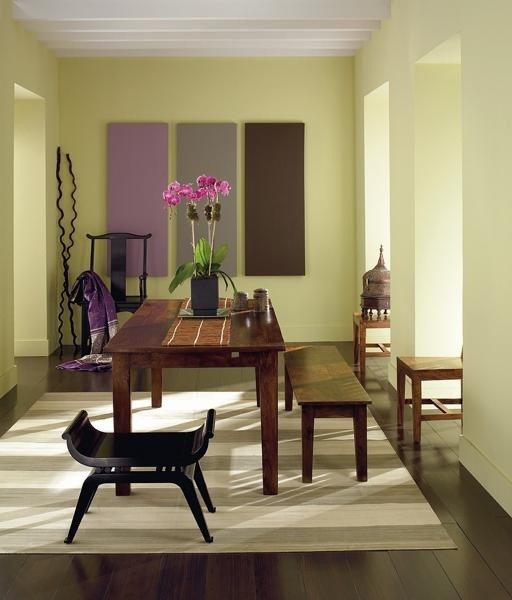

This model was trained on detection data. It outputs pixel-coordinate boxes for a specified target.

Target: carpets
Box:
[0,393,460,556]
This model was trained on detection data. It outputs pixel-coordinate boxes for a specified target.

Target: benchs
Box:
[283,345,373,483]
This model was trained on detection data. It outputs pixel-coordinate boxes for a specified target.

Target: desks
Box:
[397,356,462,444]
[353,312,391,375]
[102,297,285,495]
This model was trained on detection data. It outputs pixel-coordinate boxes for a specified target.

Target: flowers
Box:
[160,171,238,297]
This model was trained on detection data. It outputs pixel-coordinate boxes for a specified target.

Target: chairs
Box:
[75,232,153,359]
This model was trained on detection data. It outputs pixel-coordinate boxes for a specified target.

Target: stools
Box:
[61,409,216,543]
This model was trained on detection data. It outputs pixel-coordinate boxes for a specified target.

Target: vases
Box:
[191,274,218,316]
